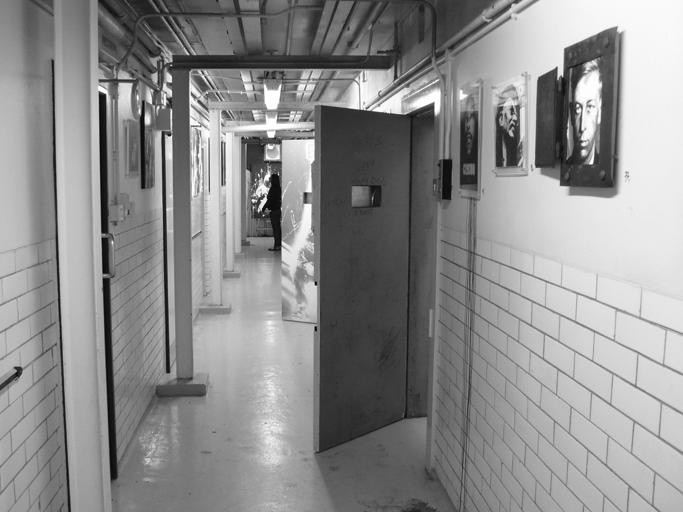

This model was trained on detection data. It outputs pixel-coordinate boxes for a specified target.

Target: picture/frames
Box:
[559,26,622,189]
[490,71,530,179]
[457,78,485,200]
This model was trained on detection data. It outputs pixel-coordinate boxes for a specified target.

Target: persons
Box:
[260,172,281,251]
[493,89,523,168]
[564,56,604,168]
[460,93,478,159]
